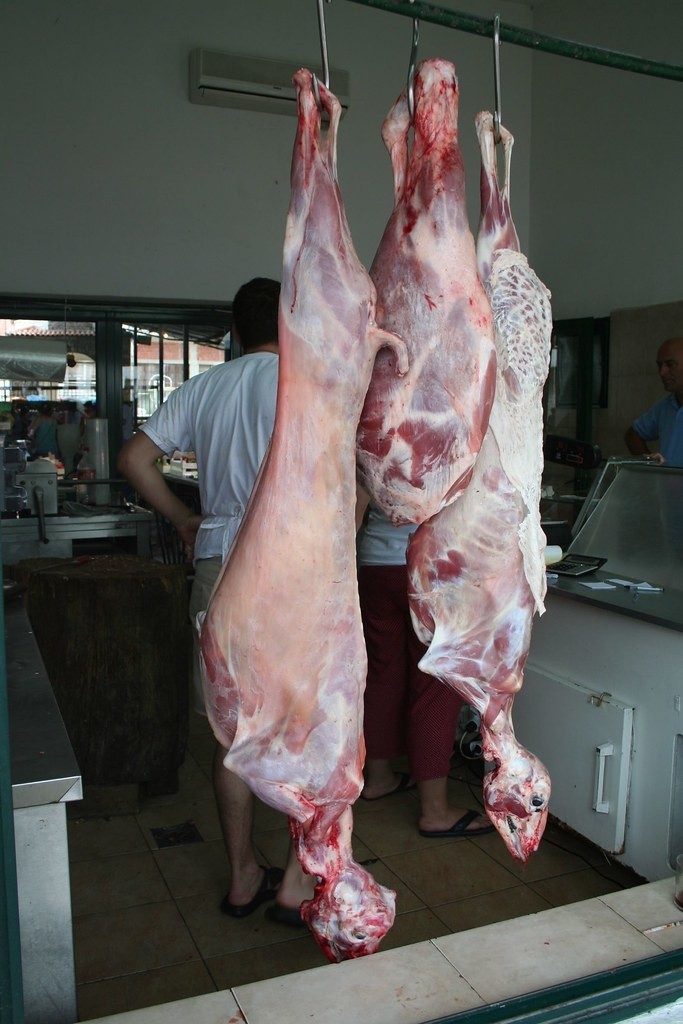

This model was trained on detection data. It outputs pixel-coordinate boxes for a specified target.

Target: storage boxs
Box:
[171,457,199,477]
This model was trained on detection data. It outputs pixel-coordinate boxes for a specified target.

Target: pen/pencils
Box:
[624,586,664,592]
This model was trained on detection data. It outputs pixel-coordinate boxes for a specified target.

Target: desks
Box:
[160,471,200,513]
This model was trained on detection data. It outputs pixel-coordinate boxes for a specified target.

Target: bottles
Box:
[77,447,96,505]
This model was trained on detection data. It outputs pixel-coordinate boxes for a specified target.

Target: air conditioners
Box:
[187,47,351,125]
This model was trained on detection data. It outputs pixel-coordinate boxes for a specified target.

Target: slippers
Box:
[359,772,418,801]
[265,895,307,927]
[420,808,496,837]
[220,867,284,918]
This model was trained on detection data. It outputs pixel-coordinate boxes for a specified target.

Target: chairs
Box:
[157,494,201,576]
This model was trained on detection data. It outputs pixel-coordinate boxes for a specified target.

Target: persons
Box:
[122,403,133,443]
[355,496,497,836]
[27,401,95,475]
[117,278,373,923]
[625,338,683,588]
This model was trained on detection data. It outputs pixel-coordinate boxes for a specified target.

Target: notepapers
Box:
[580,582,616,590]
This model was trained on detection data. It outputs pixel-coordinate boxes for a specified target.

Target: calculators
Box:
[545,553,608,577]
